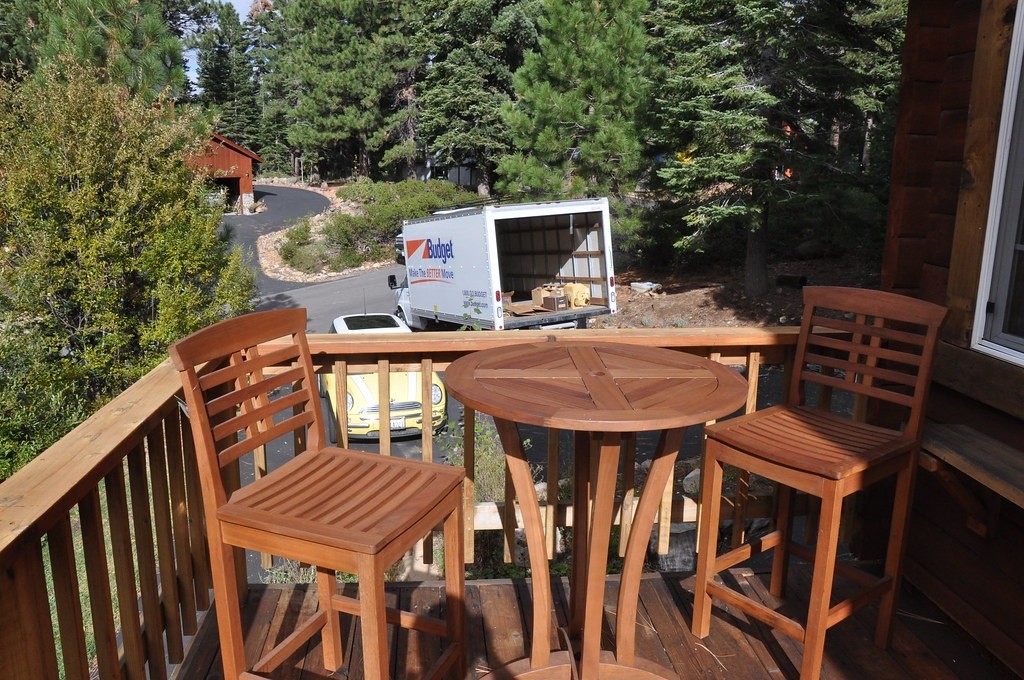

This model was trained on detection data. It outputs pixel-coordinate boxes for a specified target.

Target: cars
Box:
[318,313,447,444]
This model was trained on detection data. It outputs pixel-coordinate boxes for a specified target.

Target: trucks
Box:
[387,196,618,331]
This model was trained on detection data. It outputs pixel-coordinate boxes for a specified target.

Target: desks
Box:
[443,339,751,680]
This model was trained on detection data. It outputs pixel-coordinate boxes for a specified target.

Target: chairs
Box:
[690,284,949,679]
[166,307,469,679]
